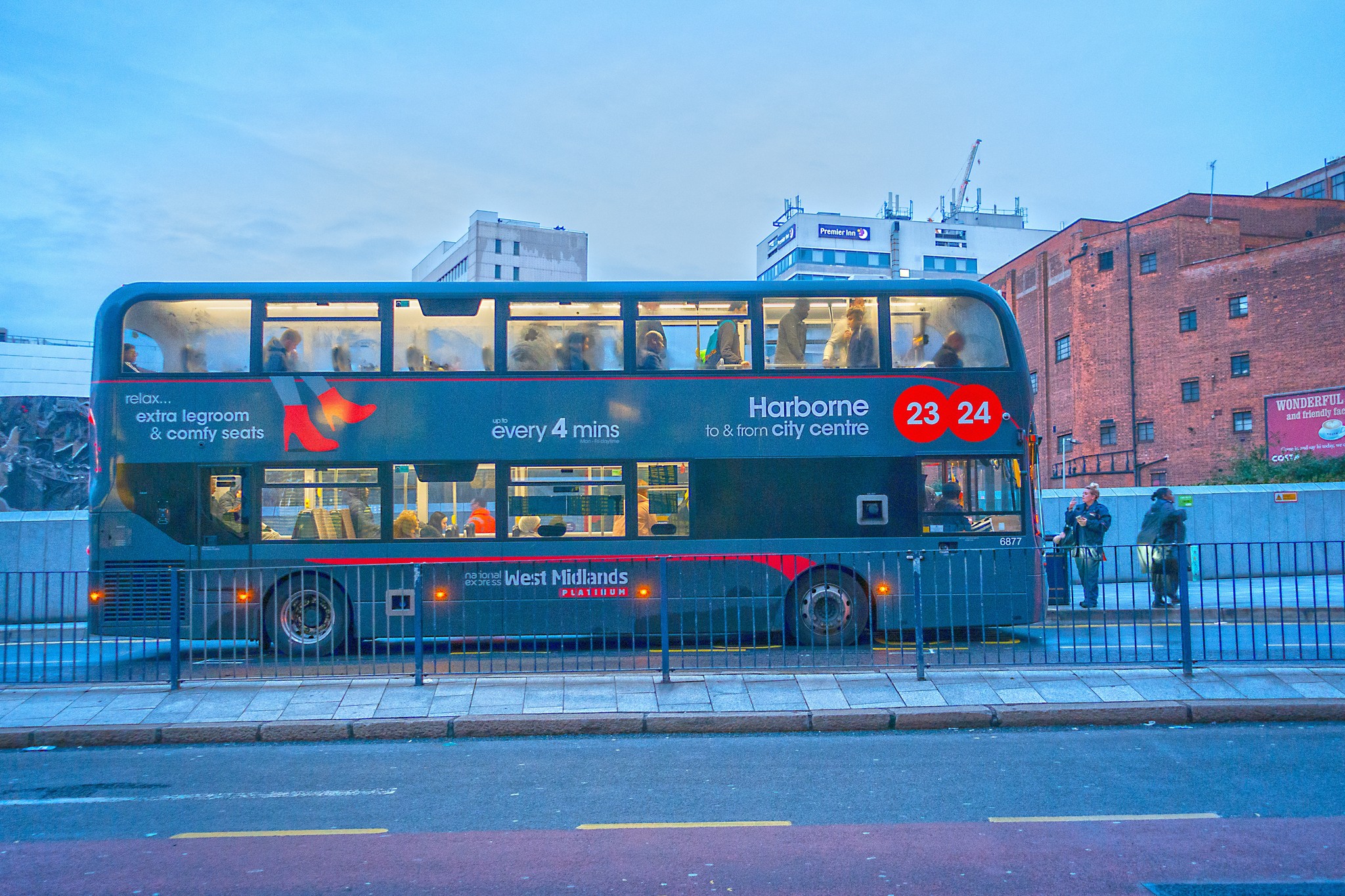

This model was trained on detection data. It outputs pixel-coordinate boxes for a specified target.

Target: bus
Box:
[75,276,1048,657]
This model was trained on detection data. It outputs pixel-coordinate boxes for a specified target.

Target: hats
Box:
[700,349,706,363]
[942,481,961,494]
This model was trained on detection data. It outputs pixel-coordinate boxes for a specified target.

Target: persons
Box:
[931,331,966,369]
[925,485,938,514]
[711,301,750,368]
[208,475,281,540]
[679,487,689,535]
[123,342,142,374]
[774,298,811,370]
[517,513,545,538]
[932,482,972,532]
[822,299,867,369]
[637,330,668,371]
[638,300,667,370]
[505,303,606,372]
[342,480,497,540]
[261,331,305,374]
[1057,482,1112,608]
[843,305,879,368]
[612,479,661,536]
[1136,488,1188,608]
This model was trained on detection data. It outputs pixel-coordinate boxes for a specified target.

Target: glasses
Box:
[129,351,139,356]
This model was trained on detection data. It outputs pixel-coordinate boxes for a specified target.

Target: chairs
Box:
[291,507,357,539]
[407,345,424,372]
[332,345,352,372]
[181,348,209,372]
[445,522,475,538]
[554,342,571,370]
[482,343,495,371]
[513,525,520,537]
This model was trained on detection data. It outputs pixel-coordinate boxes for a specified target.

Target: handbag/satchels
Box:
[1151,537,1192,573]
[1060,522,1076,551]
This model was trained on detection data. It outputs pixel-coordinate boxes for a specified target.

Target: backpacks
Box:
[705,319,736,369]
[667,494,689,535]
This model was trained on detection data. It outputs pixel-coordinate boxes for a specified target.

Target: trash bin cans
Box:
[1044,534,1072,605]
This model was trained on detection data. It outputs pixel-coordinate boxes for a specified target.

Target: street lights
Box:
[1063,436,1084,492]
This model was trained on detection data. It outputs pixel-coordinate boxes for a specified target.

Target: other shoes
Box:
[1172,597,1180,606]
[1152,600,1172,608]
[1080,601,1097,608]
